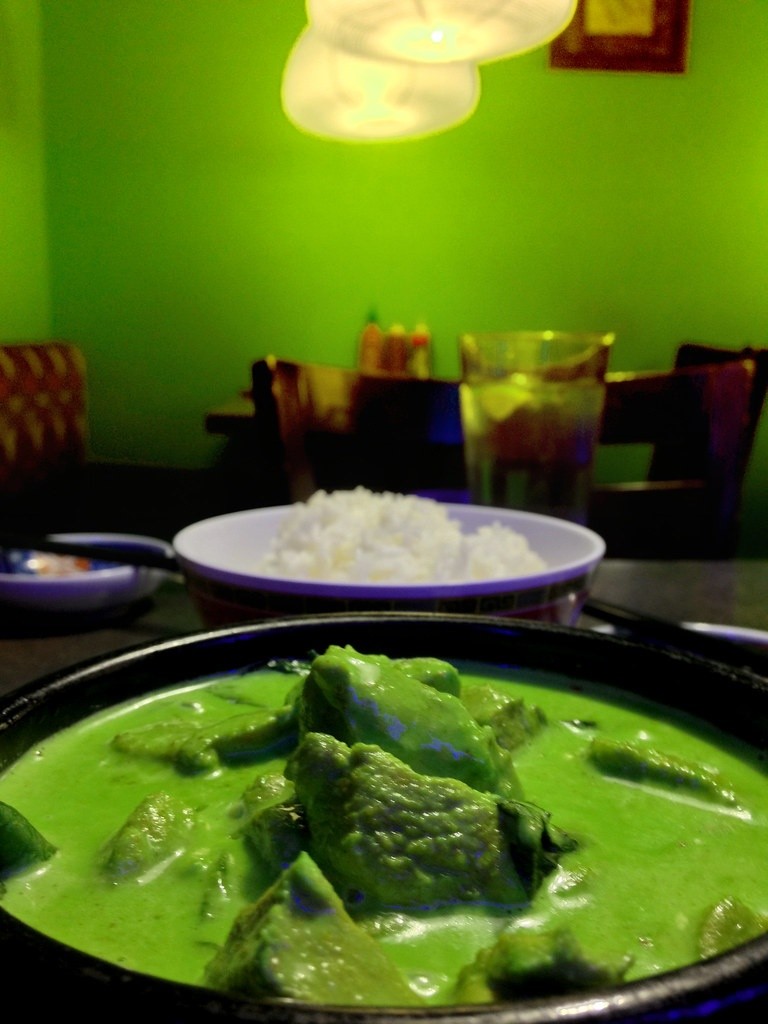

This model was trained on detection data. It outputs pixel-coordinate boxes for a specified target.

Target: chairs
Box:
[343,375,469,502]
[591,343,768,560]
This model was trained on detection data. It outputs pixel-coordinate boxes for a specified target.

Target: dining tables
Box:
[203,368,711,559]
[0,556,767,694]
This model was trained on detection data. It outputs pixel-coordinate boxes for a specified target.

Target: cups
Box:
[457,330,614,529]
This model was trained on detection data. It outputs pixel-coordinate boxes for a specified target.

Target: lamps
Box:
[279,0,580,145]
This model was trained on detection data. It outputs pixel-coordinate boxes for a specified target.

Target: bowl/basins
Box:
[171,504,605,628]
[0,610,768,1023]
[0,532,176,608]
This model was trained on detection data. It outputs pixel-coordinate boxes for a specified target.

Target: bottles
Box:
[360,312,381,375]
[409,322,434,379]
[382,320,408,377]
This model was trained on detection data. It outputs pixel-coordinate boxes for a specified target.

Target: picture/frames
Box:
[549,0,693,75]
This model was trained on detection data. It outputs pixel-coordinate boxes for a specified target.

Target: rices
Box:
[274,481,546,587]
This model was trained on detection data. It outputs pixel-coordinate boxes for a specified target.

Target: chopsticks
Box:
[580,599,768,678]
[0,534,177,572]
